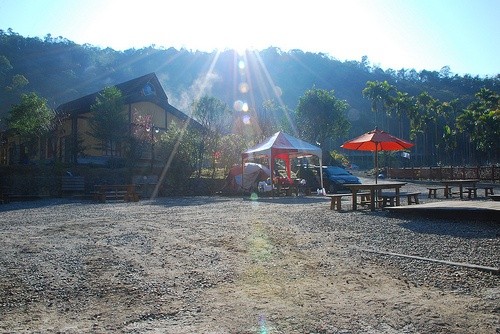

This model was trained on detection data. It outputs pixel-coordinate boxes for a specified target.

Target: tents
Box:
[241,131,323,200]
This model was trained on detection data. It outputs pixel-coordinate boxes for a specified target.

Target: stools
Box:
[328,187,495,213]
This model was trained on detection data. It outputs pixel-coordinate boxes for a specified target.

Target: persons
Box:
[297,177,311,192]
[265,178,271,185]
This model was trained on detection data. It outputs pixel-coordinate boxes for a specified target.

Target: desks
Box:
[341,182,407,211]
[441,180,480,199]
[94,184,140,202]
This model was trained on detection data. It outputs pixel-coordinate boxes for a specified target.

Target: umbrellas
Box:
[339,126,414,208]
